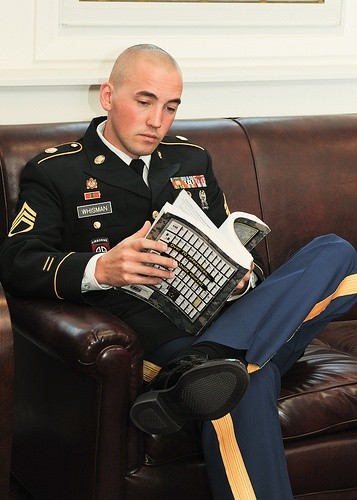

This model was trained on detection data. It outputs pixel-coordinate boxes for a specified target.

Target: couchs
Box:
[0,113,357,500]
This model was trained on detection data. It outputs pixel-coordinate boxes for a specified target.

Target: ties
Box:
[128,159,146,180]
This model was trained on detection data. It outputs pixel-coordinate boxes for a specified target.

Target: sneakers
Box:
[130,354,250,436]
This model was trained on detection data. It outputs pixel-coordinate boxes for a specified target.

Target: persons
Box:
[0,44,357,500]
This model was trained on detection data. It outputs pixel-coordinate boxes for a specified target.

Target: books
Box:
[114,189,271,337]
